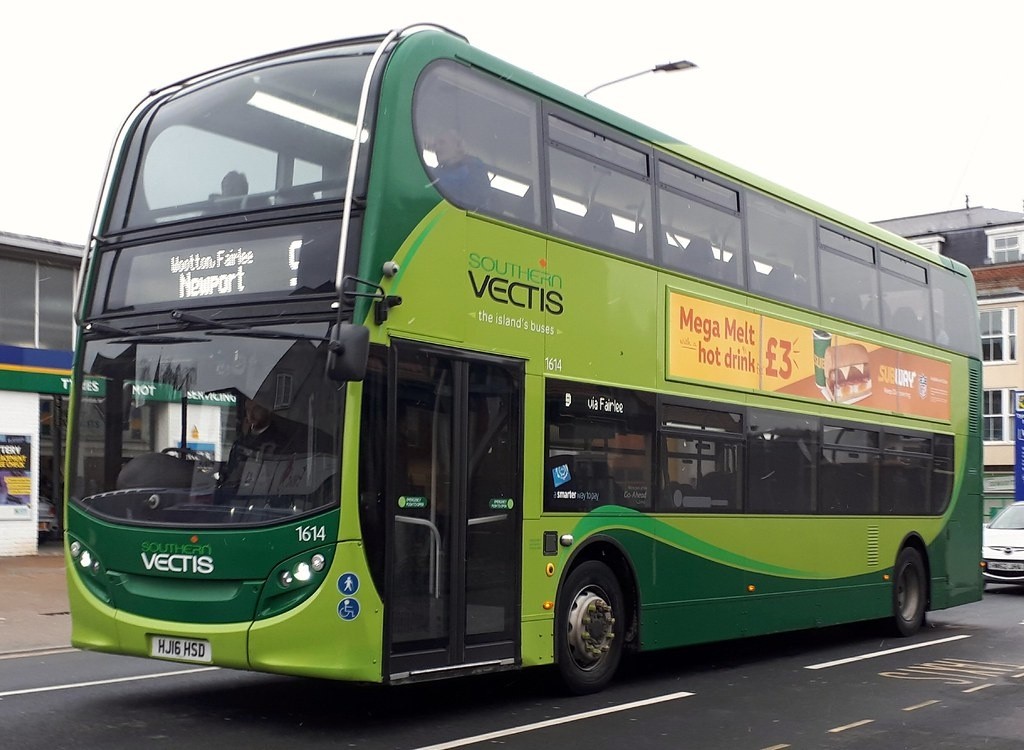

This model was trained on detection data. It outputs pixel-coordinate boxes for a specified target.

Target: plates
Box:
[821,388,873,405]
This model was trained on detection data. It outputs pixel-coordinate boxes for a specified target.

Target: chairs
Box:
[576,204,616,247]
[676,237,717,279]
[700,472,737,510]
[722,252,758,288]
[763,262,794,298]
[518,184,556,223]
[631,222,670,260]
[831,291,944,340]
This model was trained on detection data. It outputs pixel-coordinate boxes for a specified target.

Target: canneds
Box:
[811,330,833,388]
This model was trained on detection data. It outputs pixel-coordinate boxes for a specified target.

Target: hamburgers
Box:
[823,343,872,402]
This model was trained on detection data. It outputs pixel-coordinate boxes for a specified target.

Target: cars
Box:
[982,502,1024,583]
[38,496,59,546]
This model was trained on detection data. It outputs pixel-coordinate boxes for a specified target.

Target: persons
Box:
[212,391,294,509]
[201,170,272,215]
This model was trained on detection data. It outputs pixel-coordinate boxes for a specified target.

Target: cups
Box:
[813,330,831,387]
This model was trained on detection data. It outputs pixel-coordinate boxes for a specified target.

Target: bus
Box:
[64,22,984,688]
